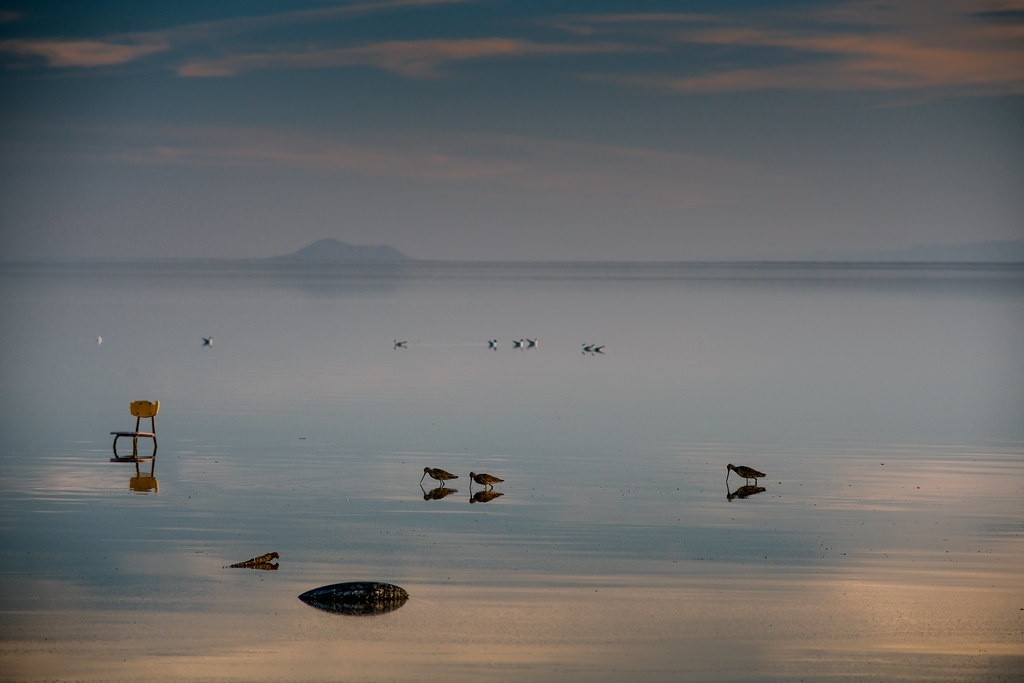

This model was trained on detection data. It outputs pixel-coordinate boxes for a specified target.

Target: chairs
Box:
[111,400,159,461]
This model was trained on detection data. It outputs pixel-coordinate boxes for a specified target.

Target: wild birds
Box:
[393,339,408,350]
[469,471,505,487]
[202,336,214,341]
[511,337,538,349]
[725,481,767,502]
[726,463,767,482]
[418,466,460,485]
[580,343,605,357]
[417,483,460,501]
[469,488,505,504]
[488,337,498,351]
